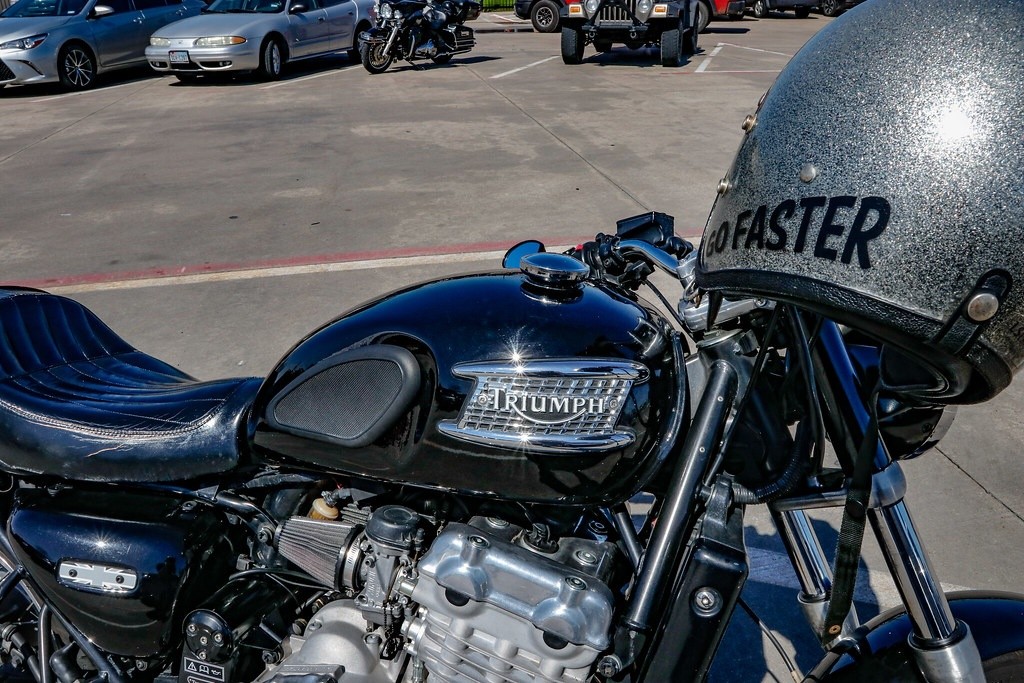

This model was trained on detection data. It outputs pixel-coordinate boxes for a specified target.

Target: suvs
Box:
[560,0,706,69]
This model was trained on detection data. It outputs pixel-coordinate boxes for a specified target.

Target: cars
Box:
[710,0,862,21]
[145,0,380,89]
[514,0,588,33]
[0,0,207,90]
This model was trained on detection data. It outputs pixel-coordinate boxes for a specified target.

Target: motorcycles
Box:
[358,0,483,76]
[0,0,1024,683]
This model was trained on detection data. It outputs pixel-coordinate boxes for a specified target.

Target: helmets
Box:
[693,0,1022,409]
